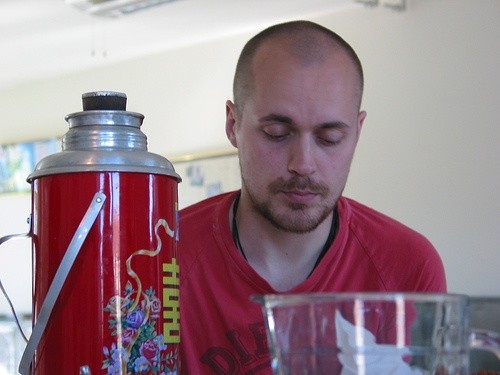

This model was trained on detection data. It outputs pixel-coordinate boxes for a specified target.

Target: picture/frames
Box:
[167,150,242,211]
[0,131,67,198]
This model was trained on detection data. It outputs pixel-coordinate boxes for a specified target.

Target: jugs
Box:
[0,88,184,374]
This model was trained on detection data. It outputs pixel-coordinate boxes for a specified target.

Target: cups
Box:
[263,288,500,375]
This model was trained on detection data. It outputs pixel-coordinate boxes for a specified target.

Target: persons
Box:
[178,20,448,375]
